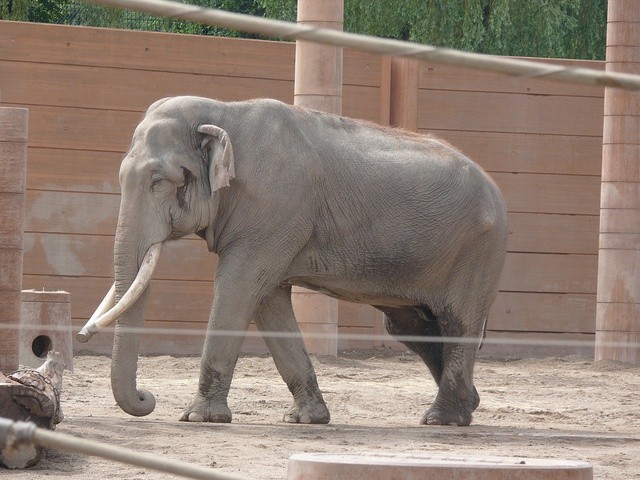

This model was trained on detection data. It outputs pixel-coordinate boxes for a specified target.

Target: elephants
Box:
[76,93,511,428]
